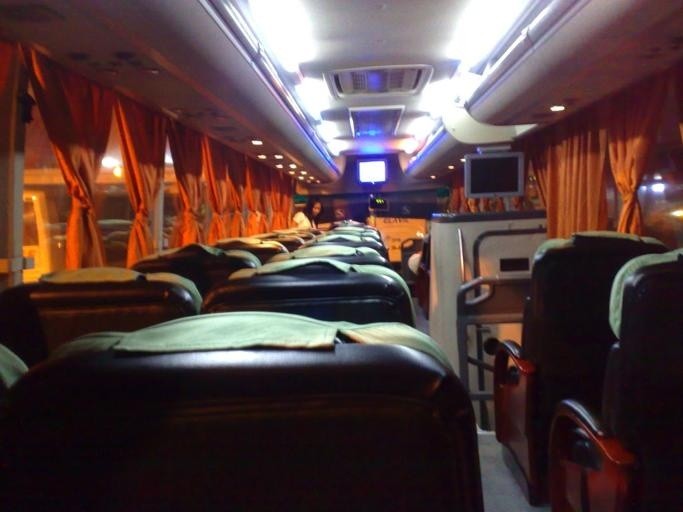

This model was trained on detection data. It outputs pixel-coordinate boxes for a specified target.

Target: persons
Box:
[289,196,324,230]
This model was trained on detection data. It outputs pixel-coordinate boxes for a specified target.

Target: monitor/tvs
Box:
[357,159,388,184]
[464,151,525,198]
